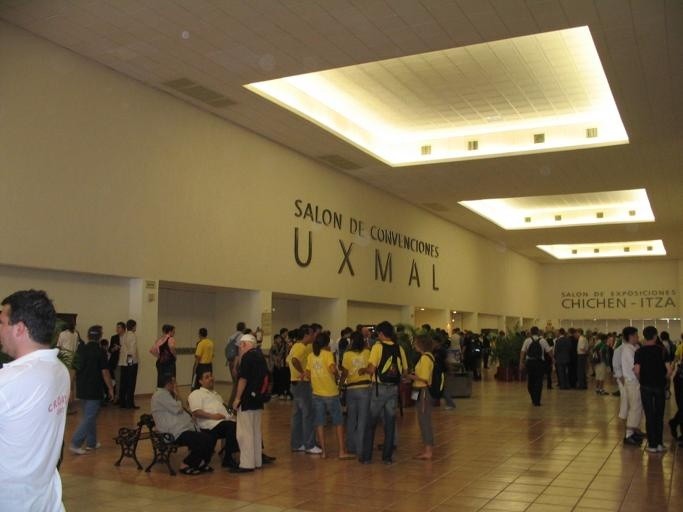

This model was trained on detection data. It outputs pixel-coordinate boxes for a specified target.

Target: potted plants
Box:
[510,320,527,382]
[485,319,515,383]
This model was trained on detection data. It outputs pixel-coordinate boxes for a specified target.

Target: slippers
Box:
[179,466,199,475]
[198,464,214,472]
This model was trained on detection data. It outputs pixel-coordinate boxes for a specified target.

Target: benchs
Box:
[111,413,153,471]
[144,410,229,477]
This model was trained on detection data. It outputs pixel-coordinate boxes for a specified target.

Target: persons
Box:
[612,326,683,452]
[517,326,621,406]
[149,321,450,475]
[0,290,70,512]
[57,315,140,454]
[450,328,505,382]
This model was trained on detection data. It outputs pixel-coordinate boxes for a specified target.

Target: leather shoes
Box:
[623,431,644,447]
[220,457,239,467]
[262,453,276,462]
[668,418,677,438]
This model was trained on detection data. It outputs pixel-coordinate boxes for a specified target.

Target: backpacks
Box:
[527,335,542,358]
[592,344,601,364]
[373,339,403,397]
[422,353,444,399]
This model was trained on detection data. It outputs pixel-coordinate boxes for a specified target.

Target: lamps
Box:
[571,246,653,254]
[414,125,601,153]
[522,210,636,224]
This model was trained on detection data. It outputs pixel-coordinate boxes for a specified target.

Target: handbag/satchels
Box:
[224,331,242,367]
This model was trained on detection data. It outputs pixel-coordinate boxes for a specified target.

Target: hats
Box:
[239,334,256,344]
[87,325,103,334]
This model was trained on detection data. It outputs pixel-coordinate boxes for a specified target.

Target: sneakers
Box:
[644,447,656,452]
[383,457,393,465]
[290,445,305,451]
[114,399,141,410]
[271,392,291,401]
[67,445,85,455]
[596,388,620,396]
[85,441,100,450]
[656,444,667,451]
[358,456,371,464]
[377,444,397,450]
[347,450,357,455]
[304,445,322,454]
[444,403,455,410]
[230,465,253,473]
